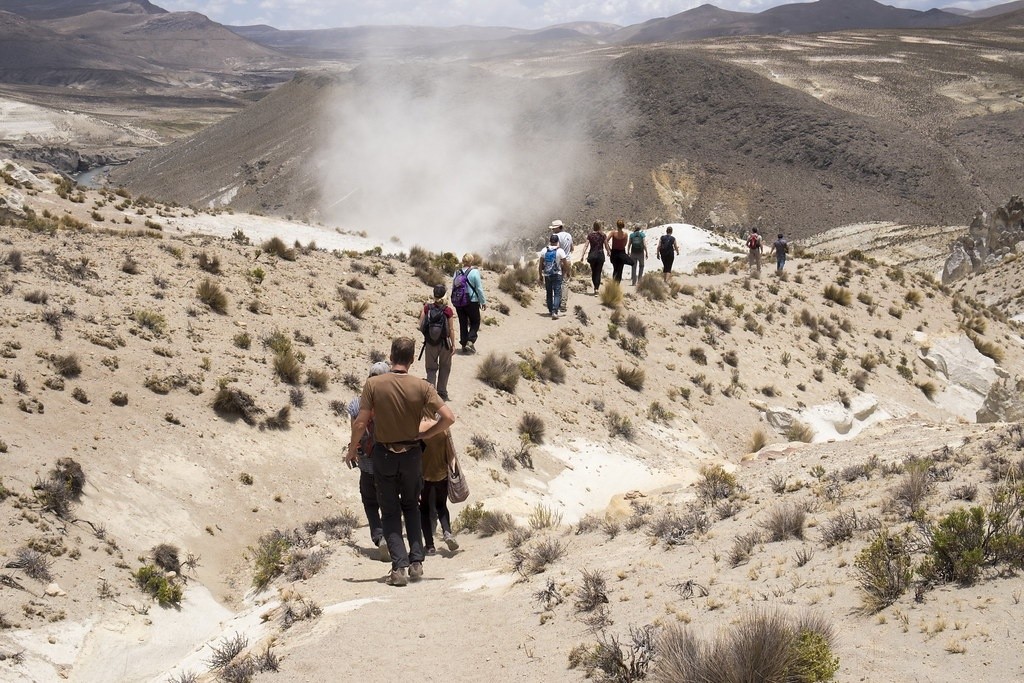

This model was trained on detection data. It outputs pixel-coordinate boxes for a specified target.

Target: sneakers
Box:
[463,347,469,353]
[385,568,407,585]
[408,562,423,576]
[466,341,476,353]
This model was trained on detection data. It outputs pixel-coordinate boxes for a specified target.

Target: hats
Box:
[549,219,563,228]
[370,361,390,377]
[433,284,448,298]
[550,235,559,243]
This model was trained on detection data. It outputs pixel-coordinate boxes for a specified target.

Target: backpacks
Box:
[749,234,760,249]
[451,268,479,306]
[418,303,449,361]
[544,246,562,274]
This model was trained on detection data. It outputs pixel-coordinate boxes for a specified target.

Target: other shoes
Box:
[423,546,435,555]
[379,540,391,561]
[552,312,558,318]
[443,531,459,550]
[594,290,600,296]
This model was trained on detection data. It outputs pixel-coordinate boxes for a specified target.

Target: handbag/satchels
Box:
[447,459,470,503]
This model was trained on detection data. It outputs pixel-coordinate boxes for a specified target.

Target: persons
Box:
[420,284,455,400]
[453,253,486,353]
[770,234,789,270]
[548,220,574,312]
[657,227,679,283]
[628,223,648,285]
[345,337,455,585]
[607,220,628,283]
[419,406,458,555]
[746,226,763,270]
[347,362,391,563]
[581,220,609,296]
[539,235,569,319]
